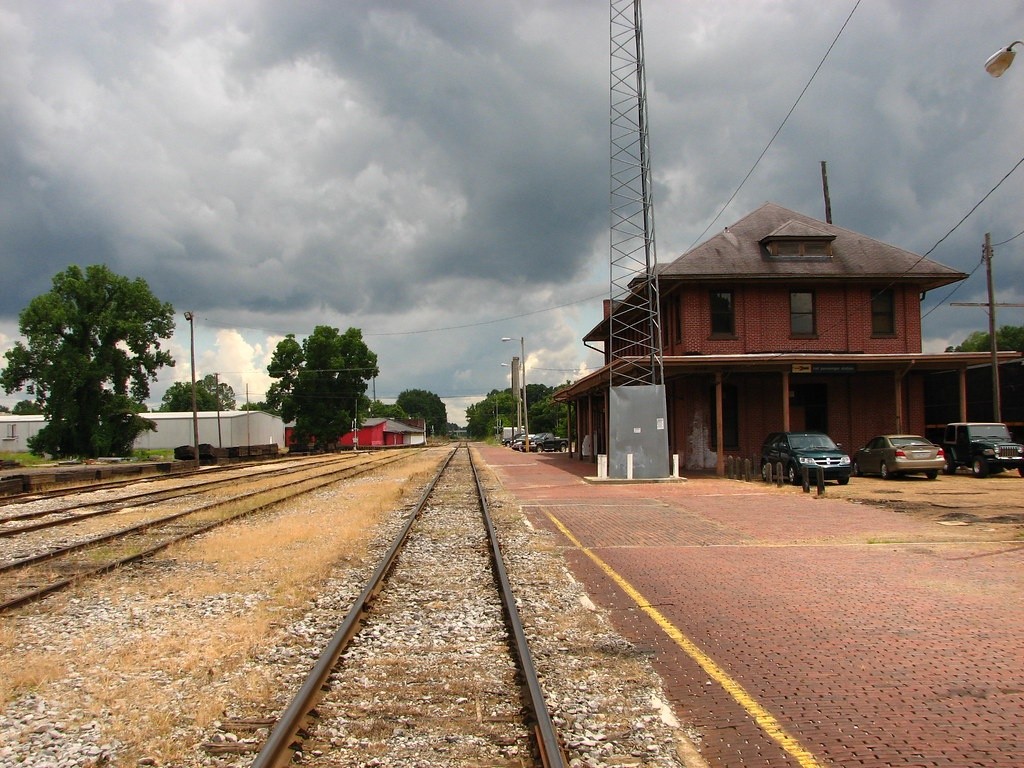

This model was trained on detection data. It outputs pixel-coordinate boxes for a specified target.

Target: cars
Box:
[852,435,946,479]
[501,433,536,451]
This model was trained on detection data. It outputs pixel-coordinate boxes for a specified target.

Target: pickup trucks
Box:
[522,433,569,453]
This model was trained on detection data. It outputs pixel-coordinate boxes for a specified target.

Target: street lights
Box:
[501,336,529,453]
[501,363,522,434]
[183,312,199,469]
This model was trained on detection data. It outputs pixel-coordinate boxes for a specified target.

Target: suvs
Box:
[943,423,1024,480]
[759,431,852,485]
